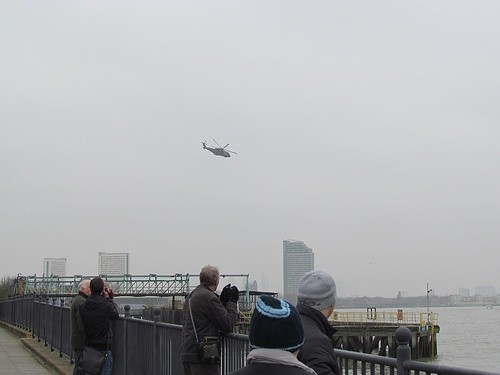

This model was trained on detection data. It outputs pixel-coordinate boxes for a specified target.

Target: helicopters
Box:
[201,138,237,157]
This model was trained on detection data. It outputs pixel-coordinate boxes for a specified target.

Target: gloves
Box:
[220,284,239,306]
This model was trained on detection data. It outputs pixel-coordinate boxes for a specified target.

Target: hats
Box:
[297,270,337,310]
[249,294,306,353]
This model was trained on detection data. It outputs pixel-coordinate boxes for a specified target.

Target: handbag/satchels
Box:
[198,336,220,365]
[101,350,113,375]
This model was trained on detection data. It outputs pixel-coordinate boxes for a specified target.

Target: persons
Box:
[71,276,119,375]
[180,265,239,375]
[295,270,340,375]
[228,294,318,375]
